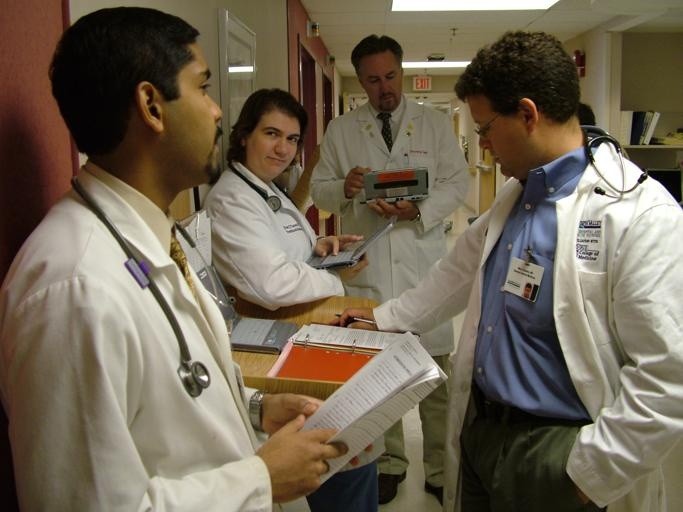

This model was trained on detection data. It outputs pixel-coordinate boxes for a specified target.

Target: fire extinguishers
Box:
[573,50,585,77]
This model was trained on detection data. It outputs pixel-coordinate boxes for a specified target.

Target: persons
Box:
[341,30,683,512]
[201,86,366,312]
[523,283,531,298]
[306,33,472,507]
[0,6,371,511]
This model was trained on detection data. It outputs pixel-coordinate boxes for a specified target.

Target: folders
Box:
[264,321,421,381]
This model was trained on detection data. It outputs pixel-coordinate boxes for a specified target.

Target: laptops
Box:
[188,265,298,355]
[307,214,397,270]
[361,167,429,204]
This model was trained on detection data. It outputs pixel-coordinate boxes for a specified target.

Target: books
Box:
[299,330,448,498]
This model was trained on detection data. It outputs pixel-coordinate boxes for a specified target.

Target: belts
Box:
[469,383,549,430]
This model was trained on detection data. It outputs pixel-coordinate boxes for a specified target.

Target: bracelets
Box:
[247,392,265,433]
[407,213,420,223]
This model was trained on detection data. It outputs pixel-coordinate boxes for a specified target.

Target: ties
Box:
[164,229,208,334]
[376,111,395,154]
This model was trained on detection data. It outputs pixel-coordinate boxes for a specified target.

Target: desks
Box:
[221,285,382,401]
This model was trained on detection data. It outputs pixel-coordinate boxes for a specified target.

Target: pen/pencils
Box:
[335,314,376,324]
